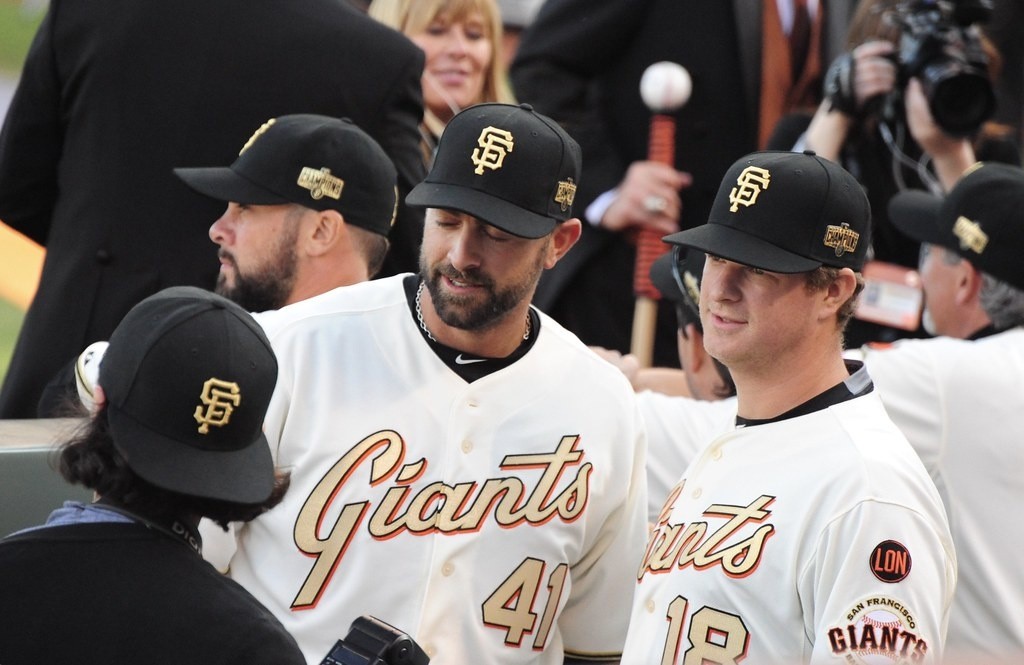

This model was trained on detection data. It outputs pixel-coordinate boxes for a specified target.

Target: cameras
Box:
[870,0,999,139]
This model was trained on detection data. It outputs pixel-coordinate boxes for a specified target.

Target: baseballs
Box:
[639,62,694,108]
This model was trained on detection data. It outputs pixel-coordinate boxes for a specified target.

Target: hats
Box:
[887,161,1024,290]
[662,148,872,274]
[406,102,582,238]
[170,113,398,235]
[99,286,278,502]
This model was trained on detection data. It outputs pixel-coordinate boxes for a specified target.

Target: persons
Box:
[74,102,635,665]
[843,163,1023,664]
[586,235,736,414]
[765,0,1003,269]
[620,149,958,665]
[0,1,425,418]
[0,286,308,665]
[365,0,518,287]
[506,0,828,347]
